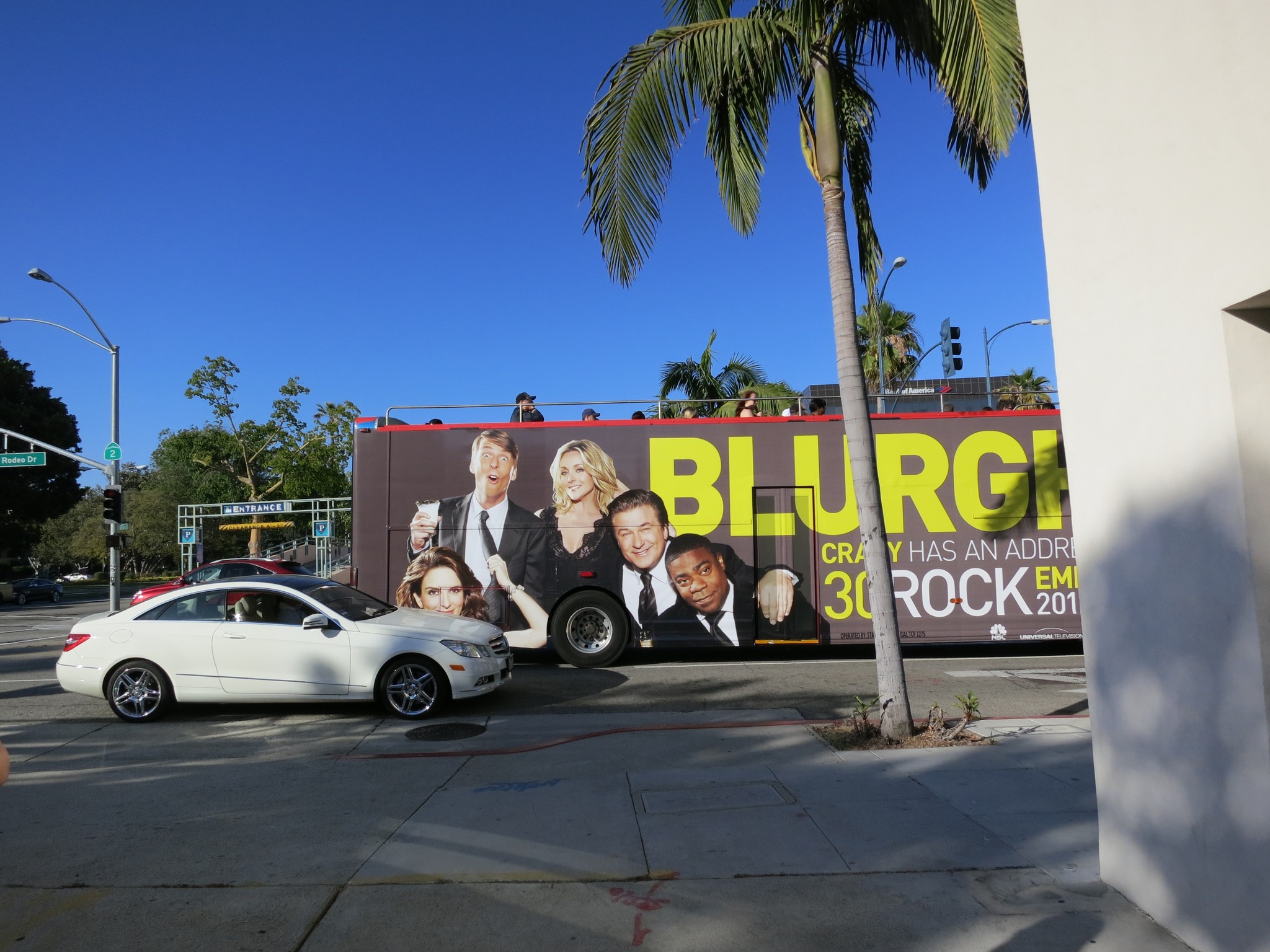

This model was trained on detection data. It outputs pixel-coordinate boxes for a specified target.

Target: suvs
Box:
[129,557,314,609]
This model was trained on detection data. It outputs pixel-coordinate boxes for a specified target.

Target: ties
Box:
[705,610,733,647]
[478,510,501,624]
[638,572,658,632]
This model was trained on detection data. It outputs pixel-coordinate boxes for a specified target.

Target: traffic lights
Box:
[103,484,122,524]
[939,316,963,380]
[106,535,120,548]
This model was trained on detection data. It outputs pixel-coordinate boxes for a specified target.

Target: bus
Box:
[350,391,1083,667]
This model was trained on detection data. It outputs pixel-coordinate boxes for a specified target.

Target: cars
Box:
[0,578,64,606]
[56,573,514,723]
[56,573,94,583]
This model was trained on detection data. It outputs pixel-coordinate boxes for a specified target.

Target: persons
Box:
[510,393,545,422]
[533,438,679,607]
[604,488,802,656]
[982,406,993,411]
[781,399,827,417]
[682,407,699,418]
[655,534,831,661]
[734,391,763,417]
[943,403,955,412]
[395,545,549,650]
[1042,403,1056,410]
[996,399,1013,411]
[582,409,601,421]
[632,411,645,419]
[408,428,544,586]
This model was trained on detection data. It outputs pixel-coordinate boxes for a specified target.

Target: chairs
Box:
[233,595,263,622]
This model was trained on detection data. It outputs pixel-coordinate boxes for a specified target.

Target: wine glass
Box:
[415,500,441,548]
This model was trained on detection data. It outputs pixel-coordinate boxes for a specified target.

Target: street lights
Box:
[874,256,908,413]
[983,319,1051,408]
[0,266,149,613]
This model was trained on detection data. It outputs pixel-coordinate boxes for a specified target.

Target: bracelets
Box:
[508,584,525,602]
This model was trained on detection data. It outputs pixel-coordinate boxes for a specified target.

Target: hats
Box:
[515,393,536,404]
[790,404,807,413]
[429,419,443,425]
[582,409,600,418]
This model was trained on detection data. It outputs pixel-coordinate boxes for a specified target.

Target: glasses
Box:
[522,399,532,402]
